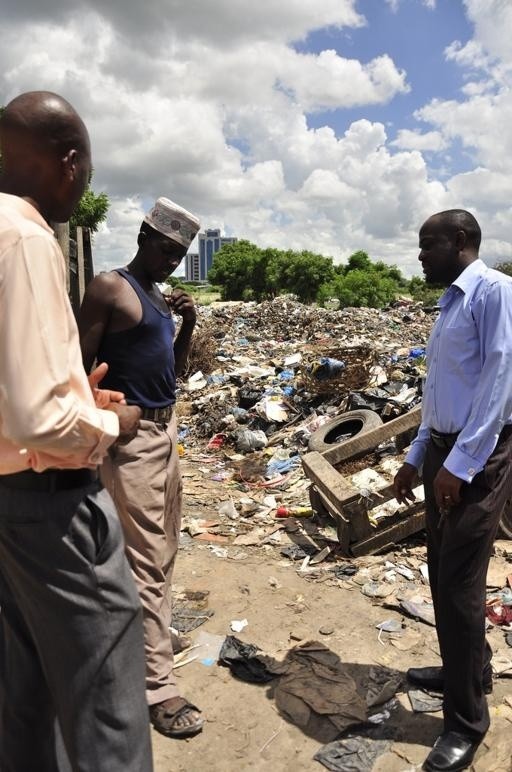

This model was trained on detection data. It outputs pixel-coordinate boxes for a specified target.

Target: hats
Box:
[143,197,201,249]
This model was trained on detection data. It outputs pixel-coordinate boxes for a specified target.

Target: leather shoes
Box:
[423,729,483,771]
[407,666,492,694]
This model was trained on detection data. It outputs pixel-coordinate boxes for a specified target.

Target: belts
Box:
[141,406,173,420]
[0,469,103,489]
[430,425,511,444]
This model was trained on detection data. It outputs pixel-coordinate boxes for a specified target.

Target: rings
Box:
[444,494,451,500]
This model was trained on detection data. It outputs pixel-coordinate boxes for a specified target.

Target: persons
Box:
[393,208,511,772]
[1,90,154,771]
[74,196,205,738]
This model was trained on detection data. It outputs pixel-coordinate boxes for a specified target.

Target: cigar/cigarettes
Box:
[437,513,445,529]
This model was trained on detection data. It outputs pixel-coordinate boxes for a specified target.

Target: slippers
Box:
[150,696,205,737]
